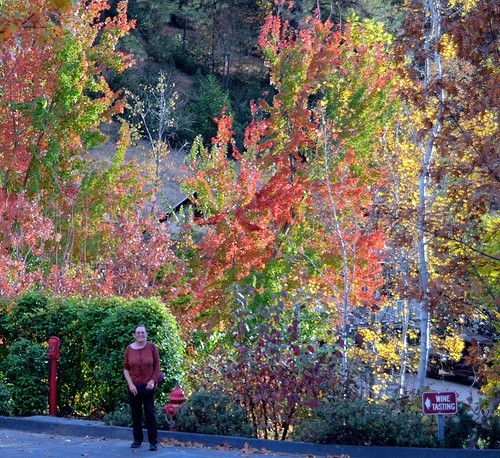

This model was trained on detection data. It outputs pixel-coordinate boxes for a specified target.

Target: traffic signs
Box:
[422,392,458,414]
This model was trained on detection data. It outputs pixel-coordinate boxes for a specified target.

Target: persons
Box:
[123,324,161,452]
[462,337,479,383]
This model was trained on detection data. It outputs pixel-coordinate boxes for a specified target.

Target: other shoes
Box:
[131,441,141,448]
[150,442,157,451]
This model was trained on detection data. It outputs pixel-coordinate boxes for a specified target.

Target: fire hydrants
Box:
[164,386,187,432]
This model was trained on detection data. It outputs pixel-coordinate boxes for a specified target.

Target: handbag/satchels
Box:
[155,370,165,388]
